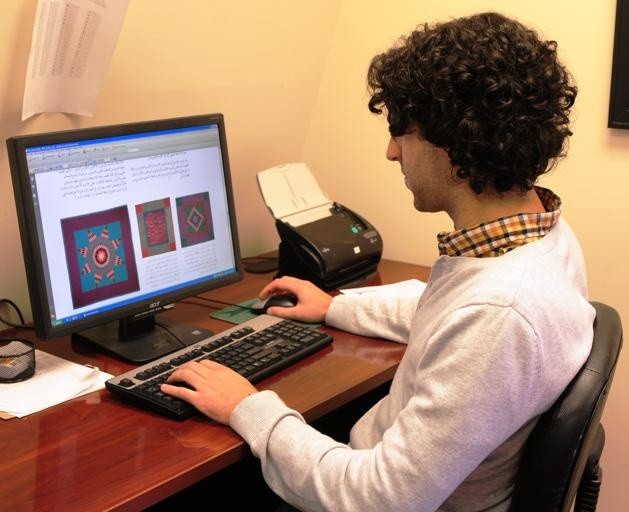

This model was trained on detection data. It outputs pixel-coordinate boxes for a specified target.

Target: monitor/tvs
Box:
[5,113,244,361]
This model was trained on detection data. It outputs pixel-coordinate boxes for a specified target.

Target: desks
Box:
[0,249,431,511]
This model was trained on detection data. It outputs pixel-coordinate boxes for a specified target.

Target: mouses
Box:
[250,294,298,314]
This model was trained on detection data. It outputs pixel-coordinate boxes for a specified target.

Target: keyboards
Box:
[105,315,333,422]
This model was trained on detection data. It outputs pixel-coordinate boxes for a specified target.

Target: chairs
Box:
[507,300,622,512]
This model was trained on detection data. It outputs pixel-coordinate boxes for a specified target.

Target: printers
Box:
[273,201,384,285]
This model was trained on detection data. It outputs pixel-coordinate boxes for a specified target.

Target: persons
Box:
[159,12,596,512]
